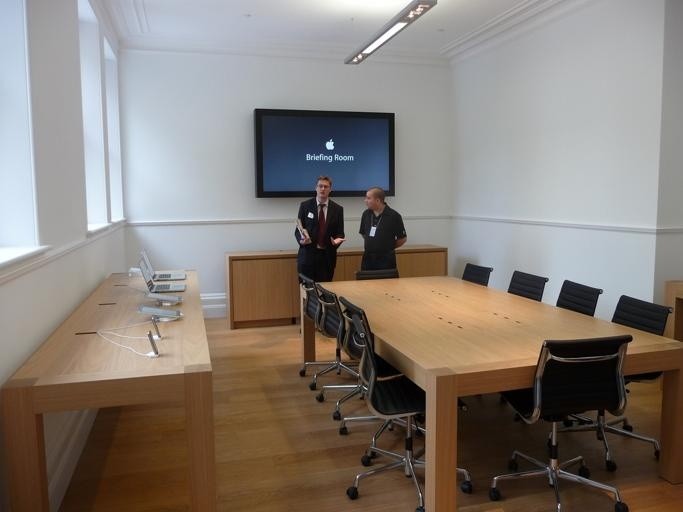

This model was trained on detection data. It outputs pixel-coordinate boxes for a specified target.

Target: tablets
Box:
[143,293,183,303]
[136,304,180,318]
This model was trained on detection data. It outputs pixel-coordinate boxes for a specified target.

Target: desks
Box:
[1,269,213,511]
[227,245,447,331]
[317,276,682,512]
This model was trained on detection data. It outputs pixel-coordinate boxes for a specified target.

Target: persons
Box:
[358,186,408,271]
[294,175,346,282]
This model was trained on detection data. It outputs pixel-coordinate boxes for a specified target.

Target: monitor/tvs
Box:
[253,109,395,199]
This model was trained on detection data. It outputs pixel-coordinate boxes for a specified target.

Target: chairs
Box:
[549,294,673,474]
[557,280,603,316]
[508,272,548,302]
[340,297,476,512]
[462,264,492,288]
[316,283,368,420]
[298,274,359,390]
[490,335,662,512]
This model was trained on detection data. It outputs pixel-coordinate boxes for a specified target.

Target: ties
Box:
[318,204,327,248]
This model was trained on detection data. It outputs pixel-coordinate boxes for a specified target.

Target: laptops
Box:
[137,256,185,293]
[138,248,185,282]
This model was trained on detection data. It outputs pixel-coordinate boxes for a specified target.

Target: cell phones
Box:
[146,331,157,355]
[151,317,161,338]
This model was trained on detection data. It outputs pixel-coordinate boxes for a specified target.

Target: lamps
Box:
[345,0,436,65]
